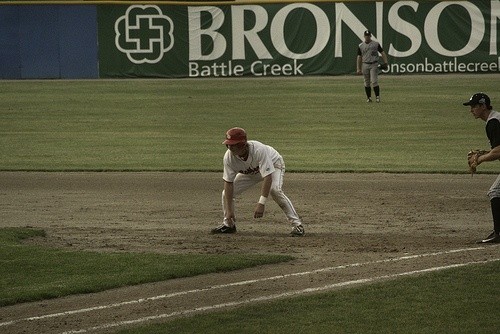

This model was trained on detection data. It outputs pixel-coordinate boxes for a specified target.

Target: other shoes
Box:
[377,97,380,102]
[366,98,372,102]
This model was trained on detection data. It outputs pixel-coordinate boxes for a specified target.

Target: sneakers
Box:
[289,224,304,237]
[476,231,500,244]
[211,224,237,234]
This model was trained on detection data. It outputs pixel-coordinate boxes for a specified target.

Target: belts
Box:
[364,61,377,64]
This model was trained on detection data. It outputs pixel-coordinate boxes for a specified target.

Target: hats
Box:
[364,30,370,35]
[463,93,490,106]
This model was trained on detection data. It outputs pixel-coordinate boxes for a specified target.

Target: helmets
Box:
[221,127,247,148]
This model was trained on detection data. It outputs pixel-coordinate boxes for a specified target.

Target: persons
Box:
[212,127,305,237]
[356,30,388,103]
[462,92,500,244]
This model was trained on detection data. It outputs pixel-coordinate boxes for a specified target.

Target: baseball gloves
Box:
[466,150,491,176]
[378,63,387,69]
